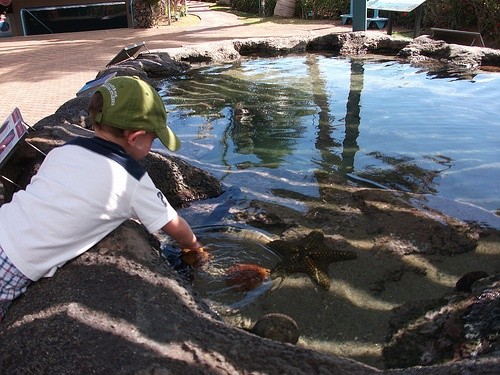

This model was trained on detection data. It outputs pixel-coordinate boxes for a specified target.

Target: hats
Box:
[86,75,182,152]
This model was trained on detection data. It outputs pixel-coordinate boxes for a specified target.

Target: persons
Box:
[0,76,201,322]
